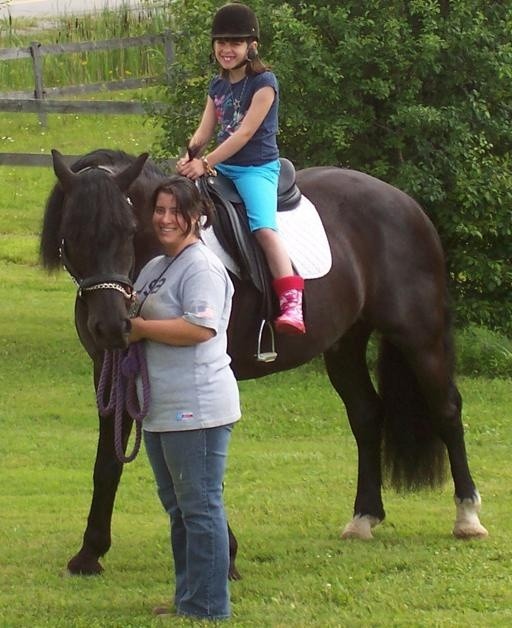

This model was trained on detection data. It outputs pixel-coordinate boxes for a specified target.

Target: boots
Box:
[273,276,306,336]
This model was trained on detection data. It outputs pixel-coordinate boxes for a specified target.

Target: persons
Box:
[174,4,306,334]
[123,177,241,619]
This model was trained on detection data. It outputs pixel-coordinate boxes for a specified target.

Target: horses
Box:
[38,144,490,578]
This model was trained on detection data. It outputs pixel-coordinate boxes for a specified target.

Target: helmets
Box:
[211,4,259,39]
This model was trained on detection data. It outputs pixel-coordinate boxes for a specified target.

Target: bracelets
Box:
[201,156,216,177]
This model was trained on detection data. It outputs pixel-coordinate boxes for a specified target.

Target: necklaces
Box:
[227,74,249,121]
